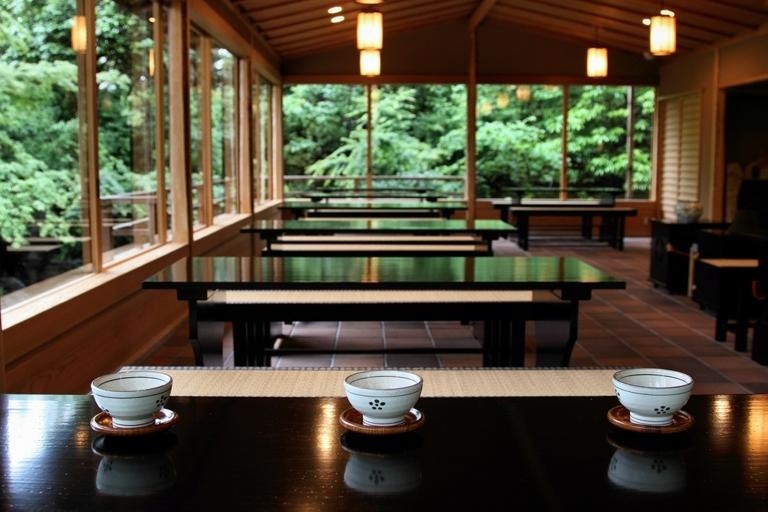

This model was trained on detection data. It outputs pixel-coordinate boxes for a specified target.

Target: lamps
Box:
[357,5,384,49]
[71,15,88,55]
[586,48,608,78]
[360,50,381,77]
[149,48,155,76]
[650,16,676,55]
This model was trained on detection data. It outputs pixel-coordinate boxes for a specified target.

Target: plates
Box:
[89,407,179,437]
[607,405,693,434]
[338,407,427,436]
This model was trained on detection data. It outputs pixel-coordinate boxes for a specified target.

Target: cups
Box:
[344,369,423,427]
[90,370,172,429]
[611,367,694,427]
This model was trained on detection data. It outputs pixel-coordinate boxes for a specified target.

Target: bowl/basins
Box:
[674,202,703,222]
